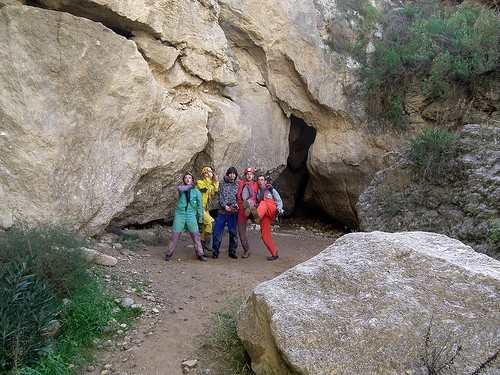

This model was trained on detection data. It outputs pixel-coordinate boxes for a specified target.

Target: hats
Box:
[183,170,195,182]
[244,166,254,175]
[226,166,238,175]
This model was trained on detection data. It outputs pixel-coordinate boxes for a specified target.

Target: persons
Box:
[223,166,273,258]
[211,166,241,259]
[164,172,207,261]
[195,165,220,257]
[253,175,284,260]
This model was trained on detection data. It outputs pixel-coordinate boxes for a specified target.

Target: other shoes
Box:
[267,255,279,261]
[243,200,251,217]
[241,249,251,259]
[228,254,238,260]
[204,231,214,251]
[212,254,219,259]
[200,239,205,251]
[197,255,207,261]
[250,207,260,224]
[165,252,173,261]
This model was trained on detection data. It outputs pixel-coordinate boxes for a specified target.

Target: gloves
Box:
[191,181,198,188]
[224,175,232,183]
[201,187,208,193]
[276,208,281,216]
[198,222,203,234]
[267,185,273,191]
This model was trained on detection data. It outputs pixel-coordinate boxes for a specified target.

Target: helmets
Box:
[201,167,212,174]
